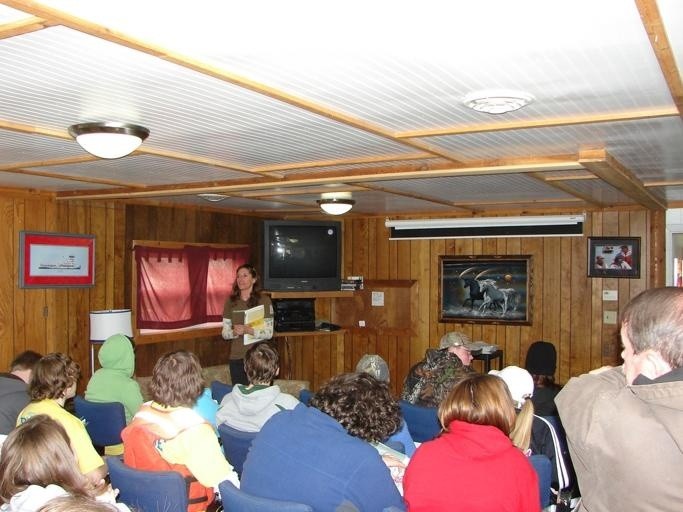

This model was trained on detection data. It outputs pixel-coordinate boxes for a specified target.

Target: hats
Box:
[356,354,391,385]
[487,365,535,408]
[439,331,483,356]
[526,341,557,375]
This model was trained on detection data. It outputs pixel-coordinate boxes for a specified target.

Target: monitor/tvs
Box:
[260,221,341,291]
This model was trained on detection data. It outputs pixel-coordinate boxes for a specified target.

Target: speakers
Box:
[274,299,315,332]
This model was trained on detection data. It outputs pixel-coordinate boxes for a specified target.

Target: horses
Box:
[462,277,500,312]
[477,278,519,317]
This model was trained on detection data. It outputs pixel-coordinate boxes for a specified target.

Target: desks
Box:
[471,350,504,374]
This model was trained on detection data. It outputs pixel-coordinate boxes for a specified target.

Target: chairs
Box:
[74,379,564,512]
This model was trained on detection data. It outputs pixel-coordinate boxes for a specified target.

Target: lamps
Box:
[67,122,150,160]
[90,309,133,376]
[316,199,355,216]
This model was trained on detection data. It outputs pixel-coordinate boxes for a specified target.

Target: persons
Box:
[356,356,420,460]
[401,330,580,512]
[1,350,138,512]
[221,263,275,385]
[553,284,682,512]
[84,336,144,426]
[124,351,240,511]
[594,246,632,269]
[240,372,403,511]
[219,343,301,432]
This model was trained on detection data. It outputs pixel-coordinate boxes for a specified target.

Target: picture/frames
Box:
[587,235,642,279]
[437,254,536,326]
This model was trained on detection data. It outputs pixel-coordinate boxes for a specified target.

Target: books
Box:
[243,304,264,346]
